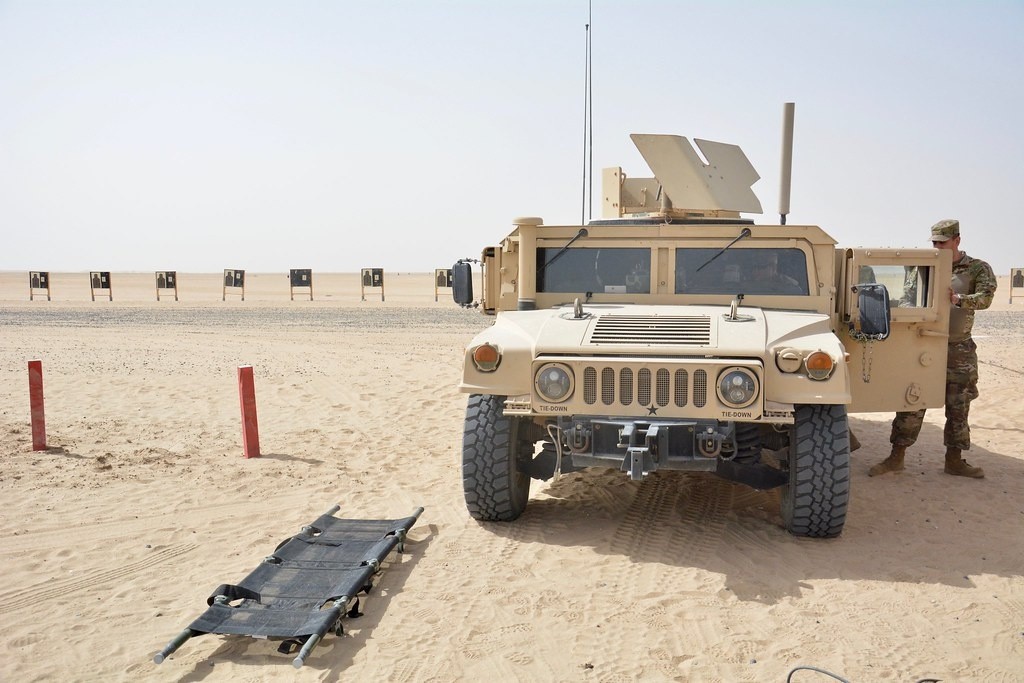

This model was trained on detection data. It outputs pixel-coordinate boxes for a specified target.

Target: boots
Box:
[868,445,907,476]
[944,447,985,478]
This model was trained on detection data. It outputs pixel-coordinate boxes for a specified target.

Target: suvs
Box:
[436,100,958,540]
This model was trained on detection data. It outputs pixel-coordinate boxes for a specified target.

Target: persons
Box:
[868,219,997,478]
[748,249,861,452]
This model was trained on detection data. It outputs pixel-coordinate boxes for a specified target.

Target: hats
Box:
[927,219,959,241]
